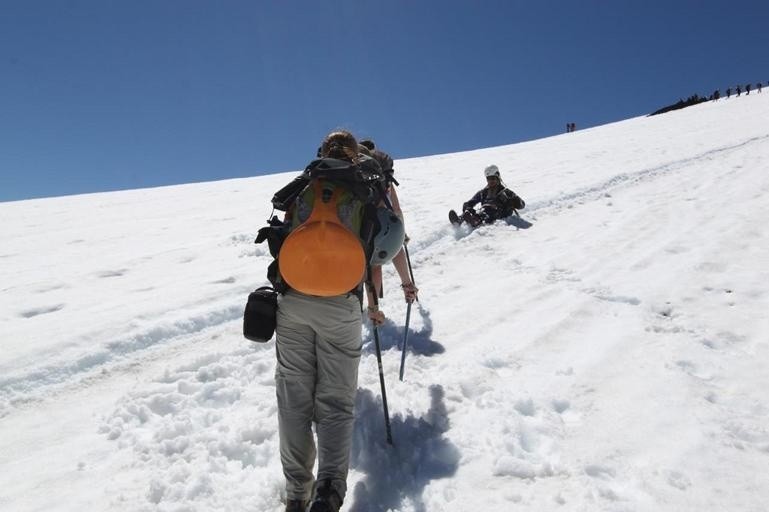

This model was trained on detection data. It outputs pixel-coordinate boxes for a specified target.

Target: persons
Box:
[677,82,769,103]
[447,163,525,228]
[355,139,417,301]
[351,150,420,311]
[570,122,575,132]
[272,131,388,510]
[566,122,570,132]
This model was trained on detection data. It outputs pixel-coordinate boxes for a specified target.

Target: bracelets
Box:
[403,281,413,288]
[367,305,380,312]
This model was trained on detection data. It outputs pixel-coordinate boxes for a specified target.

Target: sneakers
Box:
[309,477,340,512]
[286,498,306,512]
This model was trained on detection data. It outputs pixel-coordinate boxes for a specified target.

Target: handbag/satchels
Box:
[243,286,278,342]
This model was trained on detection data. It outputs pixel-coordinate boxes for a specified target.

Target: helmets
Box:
[484,165,501,187]
[369,207,405,266]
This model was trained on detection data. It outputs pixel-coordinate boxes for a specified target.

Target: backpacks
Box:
[278,178,366,297]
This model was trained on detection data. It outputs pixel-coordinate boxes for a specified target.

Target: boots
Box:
[463,212,482,228]
[449,210,463,226]
